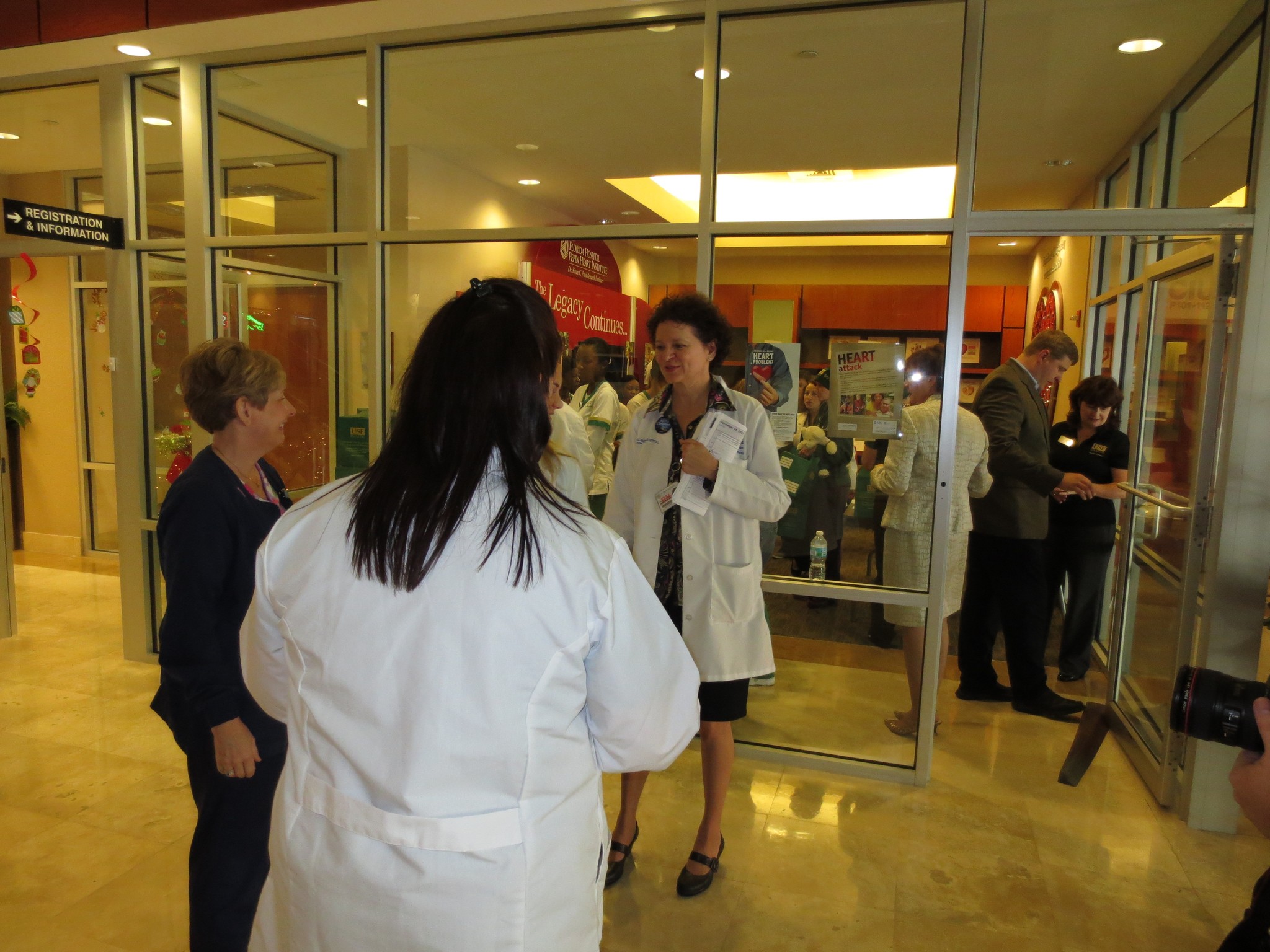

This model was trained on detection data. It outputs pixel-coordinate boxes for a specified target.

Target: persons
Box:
[955,329,1098,715]
[1216,695,1270,951]
[861,372,913,649]
[150,339,297,952]
[1040,374,1131,676]
[869,343,994,736]
[599,291,792,898]
[233,278,704,951]
[839,392,893,418]
[531,332,826,593]
[790,367,854,610]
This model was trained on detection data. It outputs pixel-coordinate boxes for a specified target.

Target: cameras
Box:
[1169,664,1270,754]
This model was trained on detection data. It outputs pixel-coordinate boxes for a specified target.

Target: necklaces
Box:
[211,445,261,487]
[902,403,910,407]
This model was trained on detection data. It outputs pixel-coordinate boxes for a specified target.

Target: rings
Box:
[225,771,234,776]
[680,458,683,464]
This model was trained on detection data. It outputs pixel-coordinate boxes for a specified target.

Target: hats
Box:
[815,367,830,389]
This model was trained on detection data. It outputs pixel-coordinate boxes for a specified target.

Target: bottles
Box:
[808,530,828,581]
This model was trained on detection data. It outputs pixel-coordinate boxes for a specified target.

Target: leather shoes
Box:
[956,681,1013,705]
[1010,689,1083,718]
[1057,663,1081,681]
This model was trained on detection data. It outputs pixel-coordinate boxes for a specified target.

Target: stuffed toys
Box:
[796,426,837,478]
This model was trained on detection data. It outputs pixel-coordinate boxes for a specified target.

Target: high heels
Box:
[879,709,940,740]
[676,832,725,897]
[602,820,639,886]
